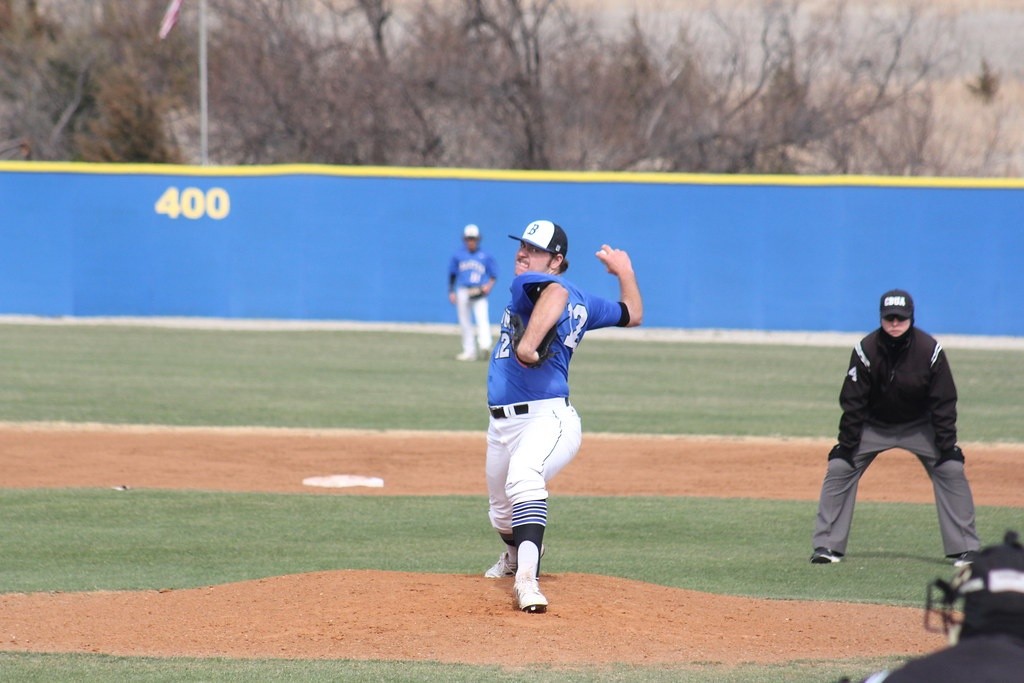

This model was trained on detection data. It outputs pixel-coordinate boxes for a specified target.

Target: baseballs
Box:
[599,250,608,264]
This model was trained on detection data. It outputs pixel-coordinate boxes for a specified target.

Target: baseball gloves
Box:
[511,314,562,370]
[468,285,486,300]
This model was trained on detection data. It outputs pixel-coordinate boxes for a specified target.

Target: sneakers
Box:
[484,542,545,577]
[513,576,549,613]
[809,547,841,563]
[945,549,979,567]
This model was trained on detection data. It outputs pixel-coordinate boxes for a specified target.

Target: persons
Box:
[809,289,980,567]
[485,220,644,614]
[448,224,497,360]
[838,530,1024,683]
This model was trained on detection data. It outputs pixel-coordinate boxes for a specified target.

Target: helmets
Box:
[946,531,1024,633]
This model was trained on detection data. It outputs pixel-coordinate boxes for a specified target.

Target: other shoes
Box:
[457,353,476,360]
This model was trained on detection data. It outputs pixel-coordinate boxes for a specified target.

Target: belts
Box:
[488,397,570,419]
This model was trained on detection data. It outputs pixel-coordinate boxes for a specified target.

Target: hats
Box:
[880,289,913,320]
[463,224,479,239]
[509,220,568,257]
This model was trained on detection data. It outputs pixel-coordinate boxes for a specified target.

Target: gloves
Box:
[827,445,856,468]
[933,446,965,468]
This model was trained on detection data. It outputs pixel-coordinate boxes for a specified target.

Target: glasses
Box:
[884,314,910,322]
